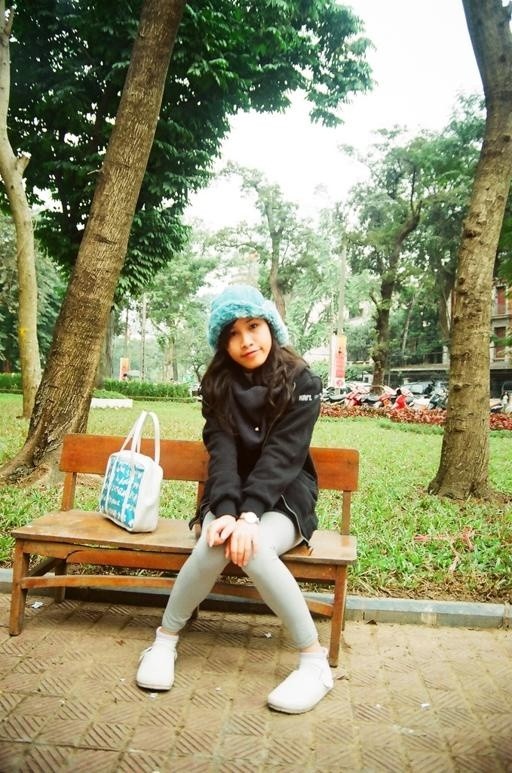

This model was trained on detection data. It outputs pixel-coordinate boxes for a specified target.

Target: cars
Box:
[320,377,511,413]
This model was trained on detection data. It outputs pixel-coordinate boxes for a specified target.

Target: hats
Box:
[207,284,289,351]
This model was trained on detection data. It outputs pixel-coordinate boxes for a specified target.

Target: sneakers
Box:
[135,648,181,690]
[266,670,333,714]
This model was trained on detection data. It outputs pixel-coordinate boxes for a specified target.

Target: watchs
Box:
[239,511,259,524]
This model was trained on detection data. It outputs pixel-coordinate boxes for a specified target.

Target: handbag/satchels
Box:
[98,409,163,534]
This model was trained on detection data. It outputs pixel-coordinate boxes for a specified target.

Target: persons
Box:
[390,389,409,410]
[121,372,127,380]
[134,280,335,720]
[499,390,509,403]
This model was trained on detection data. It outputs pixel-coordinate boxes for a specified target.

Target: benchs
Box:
[6,432,360,668]
